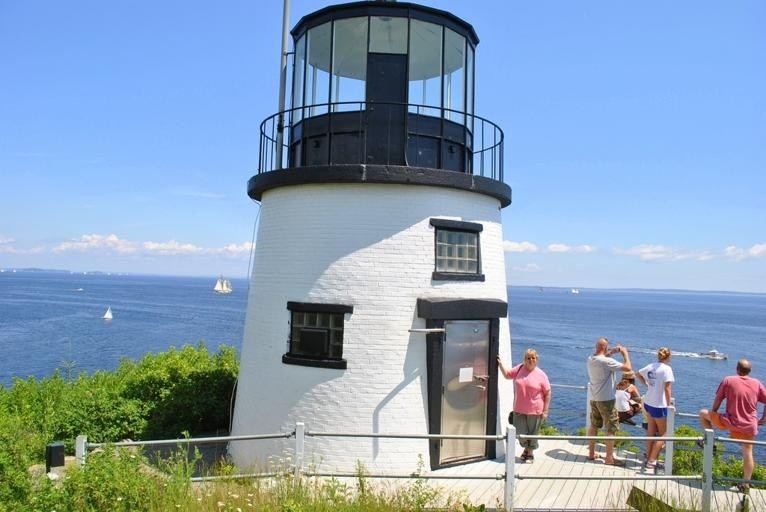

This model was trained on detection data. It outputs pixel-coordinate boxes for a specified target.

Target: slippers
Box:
[589,454,598,460]
[606,459,623,466]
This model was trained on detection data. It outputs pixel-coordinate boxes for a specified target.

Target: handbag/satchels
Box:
[508,411,513,425]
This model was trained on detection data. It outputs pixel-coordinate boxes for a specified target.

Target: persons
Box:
[637,347,675,468]
[587,338,642,467]
[495,346,552,463]
[698,359,765,492]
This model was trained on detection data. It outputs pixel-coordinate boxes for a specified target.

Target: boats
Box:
[700,348,728,360]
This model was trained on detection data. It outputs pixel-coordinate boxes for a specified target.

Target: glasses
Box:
[527,357,535,359]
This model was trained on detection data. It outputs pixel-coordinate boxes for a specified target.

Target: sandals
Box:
[521,450,533,463]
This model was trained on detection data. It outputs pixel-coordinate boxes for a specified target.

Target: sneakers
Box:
[696,440,717,456]
[643,461,664,471]
[737,484,750,494]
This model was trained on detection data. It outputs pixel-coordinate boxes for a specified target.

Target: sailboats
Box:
[213,279,233,294]
[101,305,113,321]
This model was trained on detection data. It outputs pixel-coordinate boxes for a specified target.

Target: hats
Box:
[623,371,636,379]
